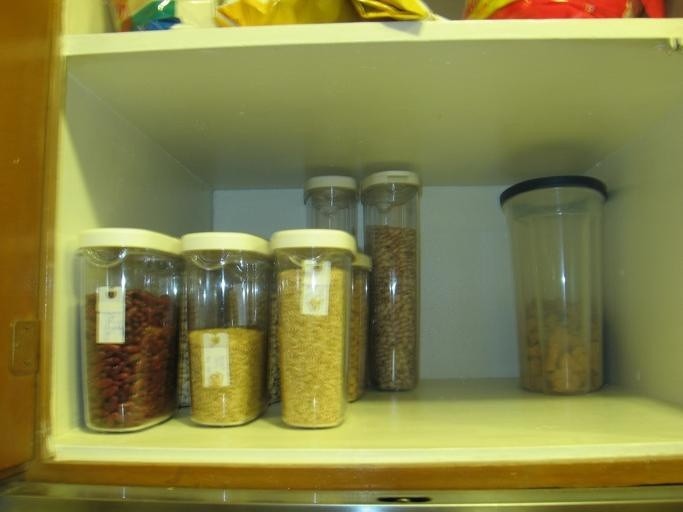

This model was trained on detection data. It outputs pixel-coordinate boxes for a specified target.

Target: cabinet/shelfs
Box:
[43,1,681,488]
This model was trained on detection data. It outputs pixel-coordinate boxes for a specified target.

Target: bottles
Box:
[305,174,359,243]
[347,253,372,402]
[361,169,420,394]
[270,229,357,430]
[499,174,609,396]
[181,230,273,428]
[77,226,184,435]
